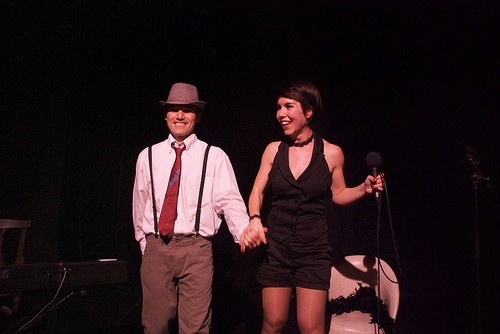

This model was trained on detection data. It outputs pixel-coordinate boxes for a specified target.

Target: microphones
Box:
[366,152,381,207]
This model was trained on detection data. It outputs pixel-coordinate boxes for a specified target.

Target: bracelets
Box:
[248,214,262,221]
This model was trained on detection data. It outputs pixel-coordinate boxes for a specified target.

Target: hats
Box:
[159,83,207,109]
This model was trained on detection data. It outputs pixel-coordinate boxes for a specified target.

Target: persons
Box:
[239,80,386,334]
[132,82,269,334]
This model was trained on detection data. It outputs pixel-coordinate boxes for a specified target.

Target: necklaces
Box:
[289,132,315,148]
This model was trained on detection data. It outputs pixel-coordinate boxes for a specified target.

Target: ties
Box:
[158,142,186,236]
